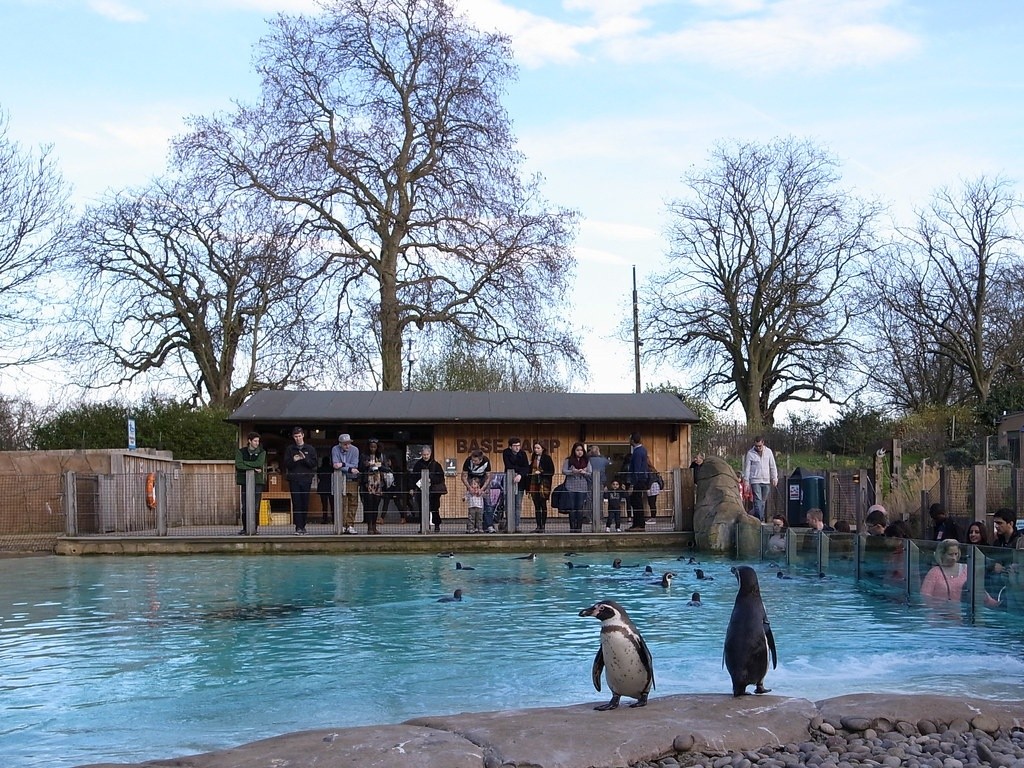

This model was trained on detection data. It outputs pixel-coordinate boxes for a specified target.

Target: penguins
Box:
[565,562,590,570]
[642,566,677,588]
[580,600,655,711]
[437,554,454,558]
[722,566,777,697]
[694,569,713,580]
[676,556,700,565]
[564,551,582,557]
[512,553,537,560]
[686,592,703,607]
[612,558,639,568]
[437,589,462,603]
[776,570,832,580]
[456,562,475,570]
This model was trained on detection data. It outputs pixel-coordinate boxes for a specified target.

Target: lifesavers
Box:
[144,472,157,506]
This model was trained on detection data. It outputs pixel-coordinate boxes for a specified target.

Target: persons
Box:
[409,445,445,534]
[331,434,360,534]
[584,446,609,525]
[689,450,705,487]
[359,439,389,535]
[235,432,266,535]
[376,454,407,524]
[732,436,1024,618]
[461,450,496,534]
[283,427,318,535]
[621,454,635,521]
[317,457,334,524]
[646,462,660,524]
[526,441,554,535]
[603,480,626,533]
[562,443,592,533]
[502,437,528,533]
[462,477,488,533]
[625,431,648,532]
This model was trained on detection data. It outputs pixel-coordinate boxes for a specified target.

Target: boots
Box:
[568,511,583,533]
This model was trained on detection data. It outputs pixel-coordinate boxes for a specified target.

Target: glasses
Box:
[292,427,304,432]
[865,524,876,529]
[754,444,762,448]
[366,439,379,443]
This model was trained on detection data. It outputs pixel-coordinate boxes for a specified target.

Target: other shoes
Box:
[530,526,545,533]
[478,529,485,533]
[342,526,382,535]
[605,527,611,533]
[238,530,246,535]
[626,517,631,521]
[616,528,622,532]
[294,527,309,535]
[466,529,474,533]
[434,525,440,531]
[377,517,386,524]
[401,518,406,524]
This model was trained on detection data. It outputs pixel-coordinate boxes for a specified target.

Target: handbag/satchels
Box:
[551,484,568,509]
[657,476,664,490]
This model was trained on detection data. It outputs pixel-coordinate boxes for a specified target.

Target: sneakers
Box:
[645,517,656,525]
[484,526,497,533]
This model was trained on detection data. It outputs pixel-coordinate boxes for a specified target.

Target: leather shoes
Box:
[625,527,645,531]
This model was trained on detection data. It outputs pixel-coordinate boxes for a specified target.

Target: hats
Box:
[338,434,353,442]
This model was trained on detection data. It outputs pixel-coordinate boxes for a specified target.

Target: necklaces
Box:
[950,574,955,578]
